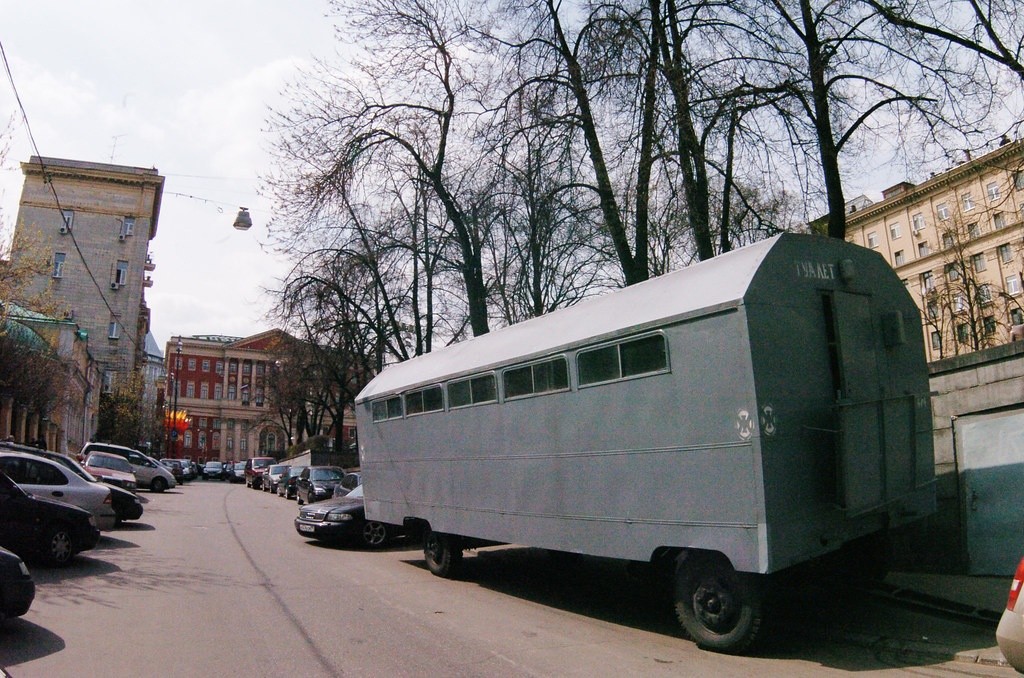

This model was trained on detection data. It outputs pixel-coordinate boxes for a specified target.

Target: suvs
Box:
[243,456,278,489]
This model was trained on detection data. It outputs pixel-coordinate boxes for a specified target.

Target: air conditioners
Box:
[110,282,119,290]
[58,227,67,234]
[118,234,125,241]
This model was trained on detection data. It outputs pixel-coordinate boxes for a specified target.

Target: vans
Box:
[74,441,177,492]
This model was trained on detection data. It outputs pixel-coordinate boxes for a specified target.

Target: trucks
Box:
[354,229,940,655]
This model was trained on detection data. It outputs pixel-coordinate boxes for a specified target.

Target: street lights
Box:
[169,335,184,459]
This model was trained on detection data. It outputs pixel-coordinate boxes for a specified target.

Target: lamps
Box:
[233,207,253,231]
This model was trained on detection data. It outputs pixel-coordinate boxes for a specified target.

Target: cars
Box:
[0,437,143,621]
[130,451,199,484]
[994,557,1024,675]
[262,464,430,547]
[197,458,247,483]
[74,451,136,494]
